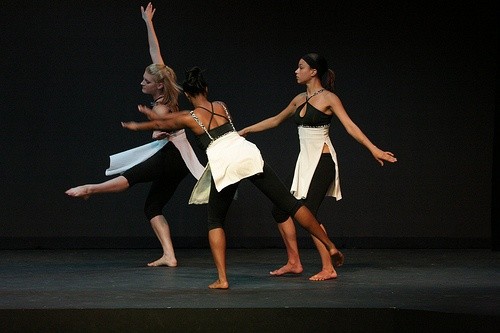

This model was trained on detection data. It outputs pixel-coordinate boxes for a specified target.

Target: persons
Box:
[65,2,206,267]
[237,55,397,279]
[121,67,344,288]
